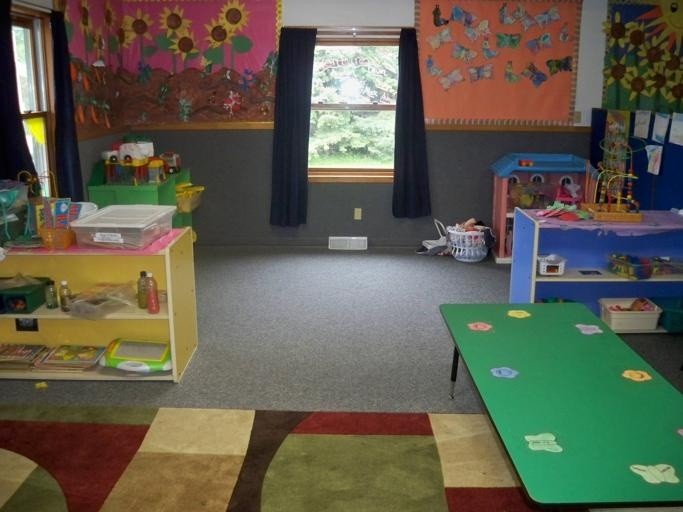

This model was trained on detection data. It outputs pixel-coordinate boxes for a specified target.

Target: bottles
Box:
[43,280,58,309]
[59,281,71,312]
[137,270,159,314]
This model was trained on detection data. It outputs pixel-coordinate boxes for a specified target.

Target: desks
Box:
[438,303,682,504]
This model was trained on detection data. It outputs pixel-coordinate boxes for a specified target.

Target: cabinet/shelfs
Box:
[87,167,193,228]
[0,227,199,386]
[489,152,600,265]
[509,207,683,335]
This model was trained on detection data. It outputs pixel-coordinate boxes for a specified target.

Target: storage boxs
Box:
[68,203,178,249]
[0,276,49,314]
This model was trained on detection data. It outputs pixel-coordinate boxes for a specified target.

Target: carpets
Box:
[0,406,683,511]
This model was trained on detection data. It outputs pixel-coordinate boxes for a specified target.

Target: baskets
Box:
[445,227,493,263]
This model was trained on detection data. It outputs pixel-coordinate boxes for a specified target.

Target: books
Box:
[0,342,107,375]
